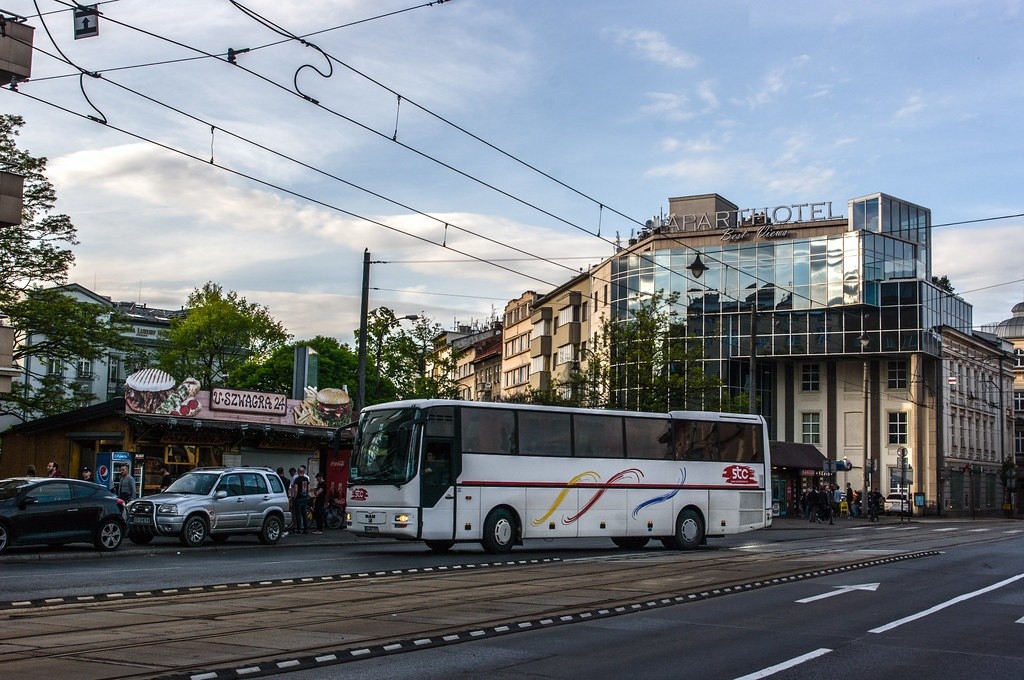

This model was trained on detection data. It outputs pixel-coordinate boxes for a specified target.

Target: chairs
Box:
[676,437,747,462]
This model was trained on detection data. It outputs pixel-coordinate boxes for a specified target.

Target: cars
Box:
[0,477,125,553]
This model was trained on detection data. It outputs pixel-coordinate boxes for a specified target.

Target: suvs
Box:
[884,493,912,516]
[126,465,292,547]
[859,492,884,514]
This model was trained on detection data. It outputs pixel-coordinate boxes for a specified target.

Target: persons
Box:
[312,472,327,535]
[869,487,886,522]
[844,482,855,517]
[118,463,136,538]
[159,464,173,493]
[26,465,37,477]
[802,483,842,525]
[276,467,291,502]
[46,461,66,496]
[82,466,96,483]
[289,465,311,536]
[424,451,436,475]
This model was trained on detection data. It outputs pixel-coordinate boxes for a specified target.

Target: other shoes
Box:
[816,518,823,524]
[303,530,309,534]
[829,523,835,525]
[311,529,322,535]
[296,530,302,534]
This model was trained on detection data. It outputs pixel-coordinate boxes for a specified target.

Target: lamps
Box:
[263,425,271,434]
[857,319,872,347]
[326,431,334,441]
[685,253,709,277]
[294,428,305,439]
[241,424,250,435]
[194,420,202,430]
[168,418,177,429]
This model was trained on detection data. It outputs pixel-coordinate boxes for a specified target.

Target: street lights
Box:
[376,315,418,385]
[570,348,596,405]
[857,329,872,518]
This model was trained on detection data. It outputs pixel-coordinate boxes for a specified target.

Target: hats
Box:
[81,465,92,472]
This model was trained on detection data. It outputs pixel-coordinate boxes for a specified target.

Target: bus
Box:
[331,399,771,555]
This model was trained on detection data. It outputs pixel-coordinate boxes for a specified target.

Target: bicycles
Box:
[295,493,343,530]
